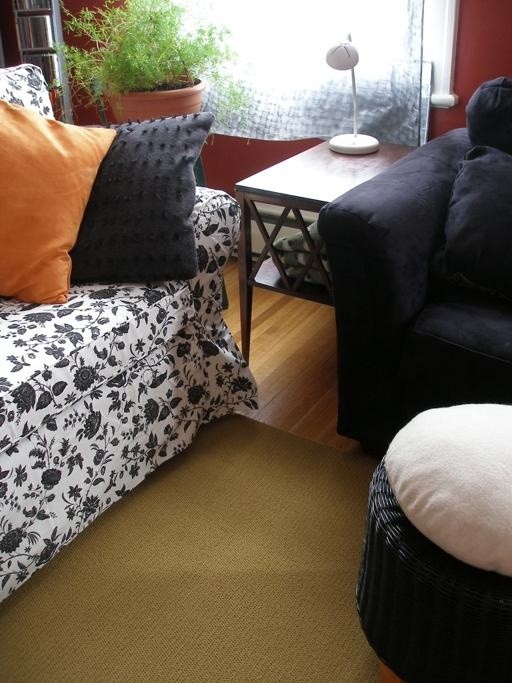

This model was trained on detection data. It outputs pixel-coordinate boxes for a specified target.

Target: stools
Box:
[353,402,512,679]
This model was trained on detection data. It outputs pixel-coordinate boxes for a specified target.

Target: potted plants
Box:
[45,0,258,145]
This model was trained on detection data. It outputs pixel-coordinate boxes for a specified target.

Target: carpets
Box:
[0,409,383,680]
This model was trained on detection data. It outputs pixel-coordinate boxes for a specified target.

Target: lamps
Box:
[326,33,380,154]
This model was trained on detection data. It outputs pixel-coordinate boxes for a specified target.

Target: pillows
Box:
[438,143,511,305]
[1,99,118,303]
[68,111,214,287]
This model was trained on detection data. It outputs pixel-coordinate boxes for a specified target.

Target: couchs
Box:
[0,60,234,604]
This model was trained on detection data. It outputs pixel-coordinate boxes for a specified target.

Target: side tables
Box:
[234,139,422,367]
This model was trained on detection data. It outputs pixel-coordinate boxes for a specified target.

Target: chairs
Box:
[317,77,512,450]
